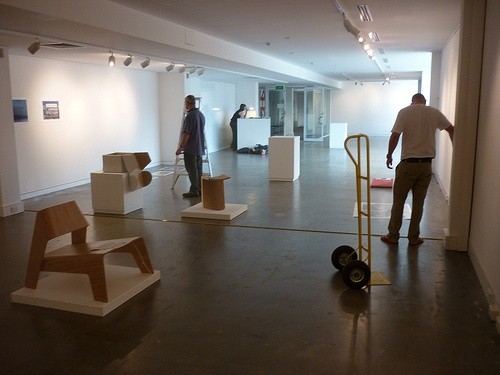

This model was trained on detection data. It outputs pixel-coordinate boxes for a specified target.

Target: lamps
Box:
[27,42,205,77]
[344,19,390,82]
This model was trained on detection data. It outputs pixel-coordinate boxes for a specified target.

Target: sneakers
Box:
[381,233,399,243]
[409,237,424,245]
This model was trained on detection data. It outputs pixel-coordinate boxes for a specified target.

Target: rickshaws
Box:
[331,133,373,289]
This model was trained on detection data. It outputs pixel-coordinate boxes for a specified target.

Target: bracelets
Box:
[386,154,392,158]
[179,145,184,147]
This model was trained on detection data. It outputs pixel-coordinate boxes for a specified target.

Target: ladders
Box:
[171,96,212,192]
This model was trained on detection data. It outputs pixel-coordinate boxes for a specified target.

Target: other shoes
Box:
[182,191,200,197]
[231,145,234,148]
[232,146,236,149]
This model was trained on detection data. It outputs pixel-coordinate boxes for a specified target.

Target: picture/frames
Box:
[43,101,59,119]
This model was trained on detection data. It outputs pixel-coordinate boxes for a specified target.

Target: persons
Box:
[381,94,454,245]
[175,95,205,198]
[230,104,249,150]
